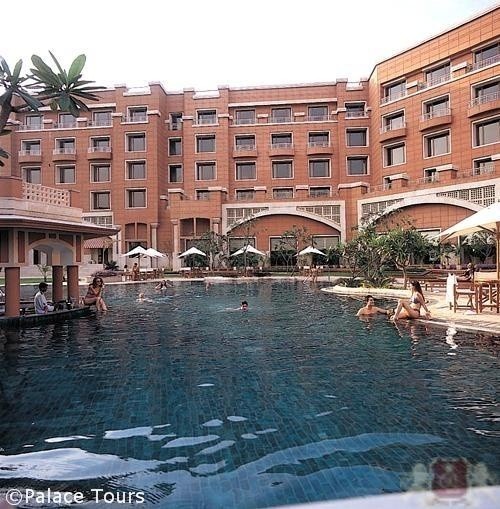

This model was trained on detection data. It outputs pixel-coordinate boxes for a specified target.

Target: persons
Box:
[308,274,315,282]
[311,263,319,283]
[156,280,174,289]
[137,294,146,300]
[33,283,55,314]
[390,279,432,323]
[241,301,249,312]
[133,262,138,276]
[437,262,473,281]
[84,277,108,310]
[123,264,135,281]
[356,295,391,316]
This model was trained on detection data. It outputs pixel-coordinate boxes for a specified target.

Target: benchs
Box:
[404,266,500,314]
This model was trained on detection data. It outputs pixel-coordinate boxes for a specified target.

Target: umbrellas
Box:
[119,245,157,268]
[294,245,327,264]
[135,247,164,267]
[178,246,211,269]
[429,202,500,281]
[230,244,266,267]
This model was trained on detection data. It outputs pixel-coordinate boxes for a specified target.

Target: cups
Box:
[67,302,71,309]
